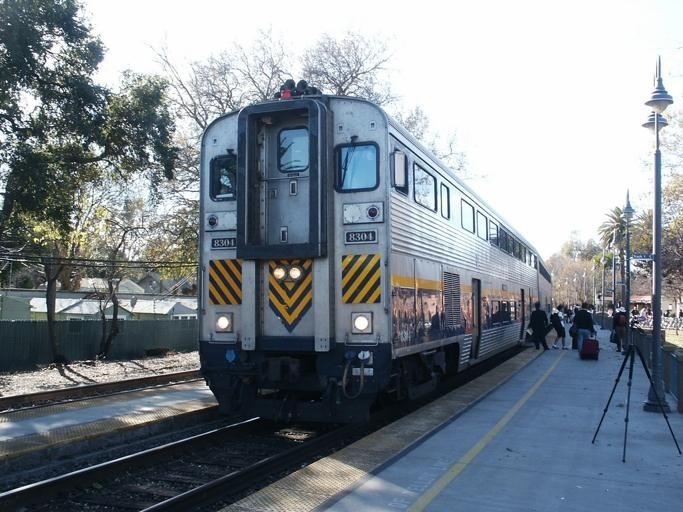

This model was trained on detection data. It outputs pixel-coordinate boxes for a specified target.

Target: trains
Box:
[197,78,554,426]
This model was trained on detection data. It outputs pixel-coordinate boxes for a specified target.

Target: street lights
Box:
[640,54,674,414]
[619,188,637,355]
[599,227,619,331]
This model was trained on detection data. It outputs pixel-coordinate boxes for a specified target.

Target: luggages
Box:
[580,331,599,360]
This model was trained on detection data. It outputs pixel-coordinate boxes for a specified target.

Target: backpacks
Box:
[550,312,562,328]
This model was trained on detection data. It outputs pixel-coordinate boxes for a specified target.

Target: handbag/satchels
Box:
[610,329,616,343]
[569,324,576,336]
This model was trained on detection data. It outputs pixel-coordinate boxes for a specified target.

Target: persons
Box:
[526,300,683,355]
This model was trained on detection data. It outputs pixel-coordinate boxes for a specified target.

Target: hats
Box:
[615,307,626,313]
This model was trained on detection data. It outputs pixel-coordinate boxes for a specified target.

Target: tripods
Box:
[591,343,682,463]
